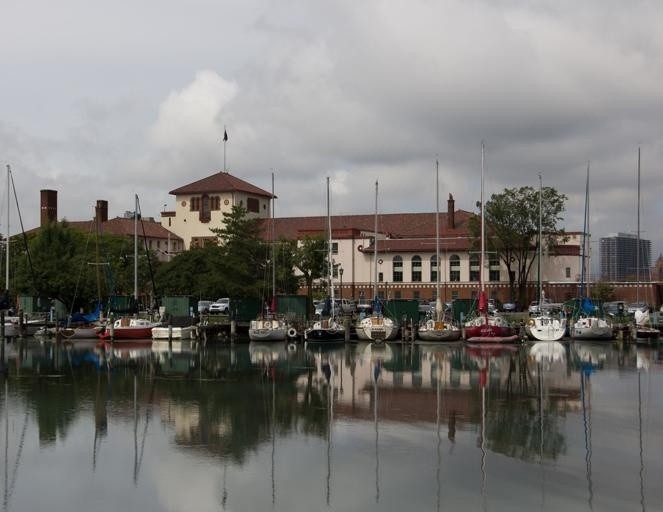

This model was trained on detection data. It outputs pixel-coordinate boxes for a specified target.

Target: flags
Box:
[222,129,227,142]
